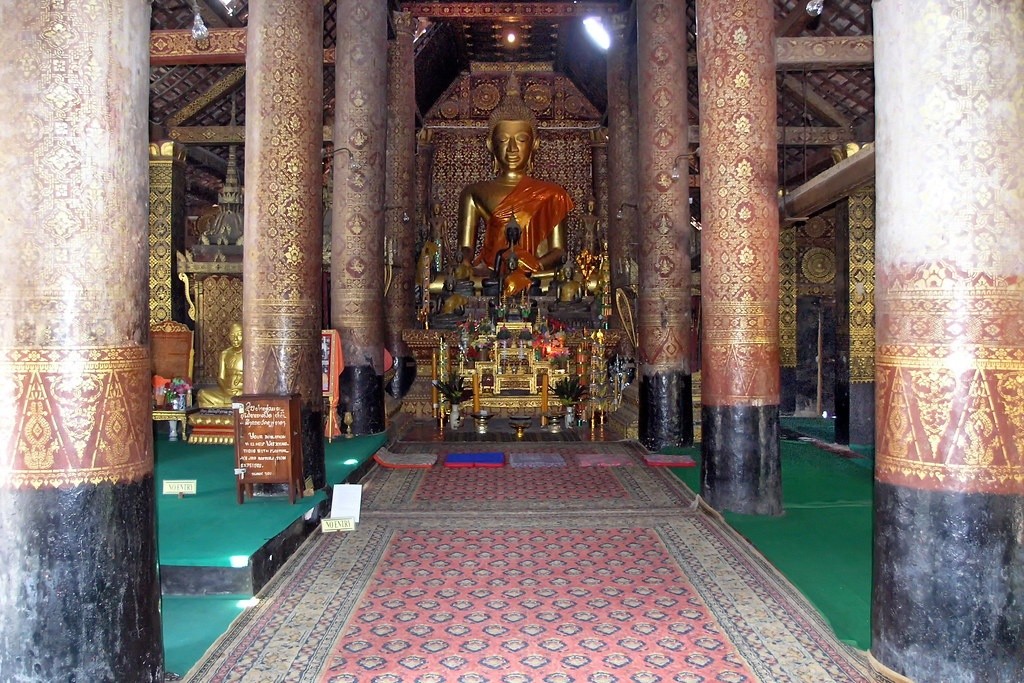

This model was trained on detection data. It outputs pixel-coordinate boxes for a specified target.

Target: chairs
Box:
[149,318,198,441]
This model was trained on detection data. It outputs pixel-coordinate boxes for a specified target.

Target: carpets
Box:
[176,438,913,683]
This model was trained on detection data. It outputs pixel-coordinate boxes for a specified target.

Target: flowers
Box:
[458,346,480,362]
[456,321,474,333]
[546,346,571,366]
[470,337,491,351]
[519,325,532,340]
[171,375,193,393]
[496,322,511,340]
[530,329,560,347]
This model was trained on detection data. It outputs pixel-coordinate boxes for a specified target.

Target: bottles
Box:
[171,392,180,410]
[166,389,172,403]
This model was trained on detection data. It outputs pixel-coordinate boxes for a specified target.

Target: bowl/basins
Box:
[157,404,172,410]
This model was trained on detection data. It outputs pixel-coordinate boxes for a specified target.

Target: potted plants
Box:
[547,373,592,431]
[432,372,477,430]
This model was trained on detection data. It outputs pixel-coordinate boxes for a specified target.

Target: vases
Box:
[541,347,547,359]
[479,348,488,360]
[177,393,188,410]
[460,332,470,348]
[558,364,567,373]
[500,340,508,348]
[466,362,474,369]
[521,340,528,347]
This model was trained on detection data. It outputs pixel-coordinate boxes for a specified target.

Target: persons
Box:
[482,206,541,290]
[197,322,243,408]
[430,64,602,296]
[575,193,603,255]
[548,250,592,313]
[430,267,468,328]
[450,243,475,294]
[549,248,586,288]
[427,198,450,273]
[599,239,609,270]
[488,239,537,310]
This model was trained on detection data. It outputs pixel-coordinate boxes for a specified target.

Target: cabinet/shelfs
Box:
[229,392,303,505]
